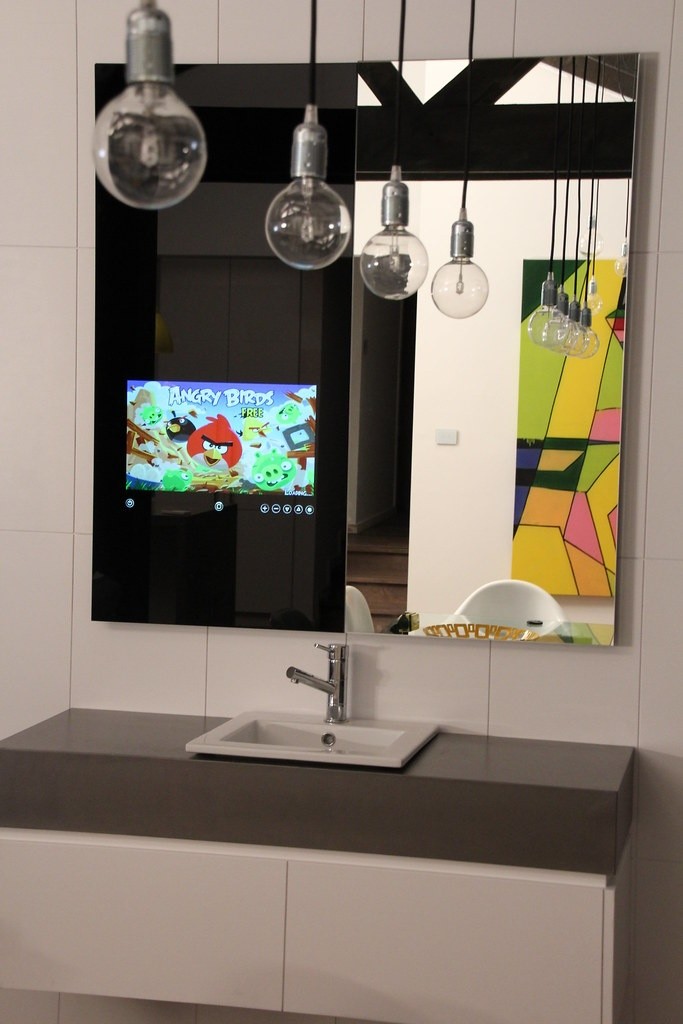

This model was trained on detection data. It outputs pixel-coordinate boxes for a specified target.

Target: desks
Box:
[408,621,614,647]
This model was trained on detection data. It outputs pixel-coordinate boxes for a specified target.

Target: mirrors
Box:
[344,51,640,648]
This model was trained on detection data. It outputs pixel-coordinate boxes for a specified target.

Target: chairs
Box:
[345,585,374,632]
[453,579,567,620]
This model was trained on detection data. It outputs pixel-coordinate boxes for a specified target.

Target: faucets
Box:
[286,643,350,724]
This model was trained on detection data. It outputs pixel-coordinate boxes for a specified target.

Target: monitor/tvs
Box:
[119,378,321,522]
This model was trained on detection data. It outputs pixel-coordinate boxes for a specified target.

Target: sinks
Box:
[185,710,439,768]
